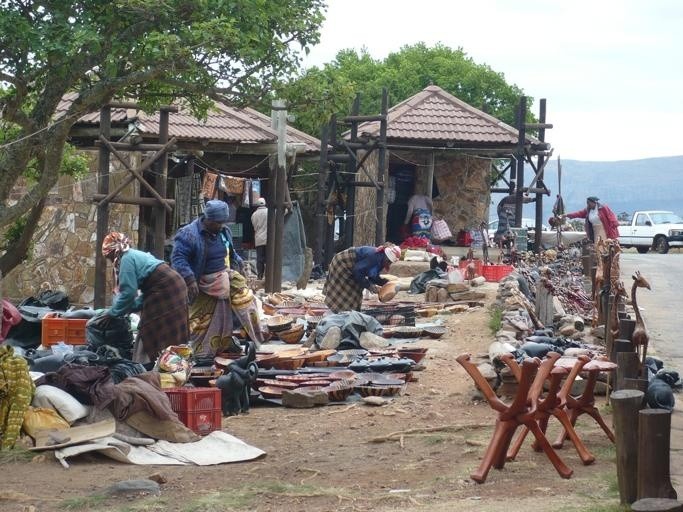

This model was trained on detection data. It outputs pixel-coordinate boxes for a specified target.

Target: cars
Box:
[488,218,546,246]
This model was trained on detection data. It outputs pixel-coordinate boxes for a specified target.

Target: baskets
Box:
[162,387,221,436]
[41,318,87,347]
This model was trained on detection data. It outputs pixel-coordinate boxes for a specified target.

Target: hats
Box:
[383,244,401,264]
[204,200,229,222]
[257,198,267,206]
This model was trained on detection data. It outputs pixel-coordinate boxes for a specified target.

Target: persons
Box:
[496,186,537,249]
[322,243,401,316]
[92,231,188,369]
[561,197,620,246]
[403,185,434,241]
[250,198,269,280]
[169,199,272,364]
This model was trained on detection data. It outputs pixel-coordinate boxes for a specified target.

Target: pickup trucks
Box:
[618,211,683,254]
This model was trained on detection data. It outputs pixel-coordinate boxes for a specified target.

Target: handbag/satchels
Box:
[429,219,452,245]
[31,289,69,310]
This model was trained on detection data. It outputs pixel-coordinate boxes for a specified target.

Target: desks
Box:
[542,358,618,449]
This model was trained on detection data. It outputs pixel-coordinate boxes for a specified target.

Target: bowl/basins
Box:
[255,347,429,402]
[268,299,477,344]
